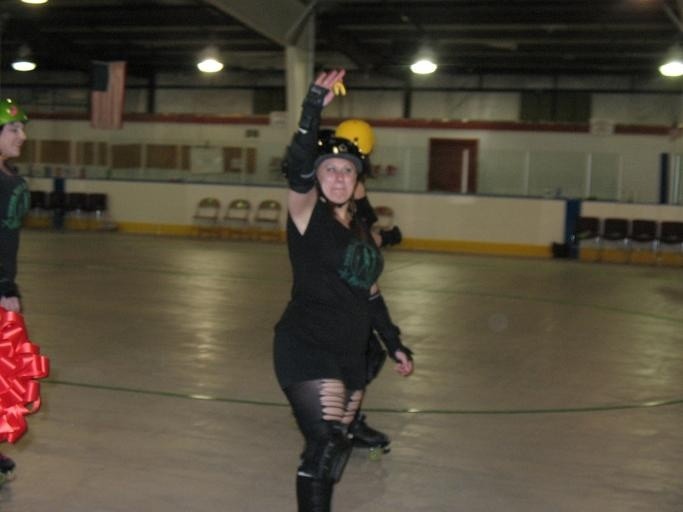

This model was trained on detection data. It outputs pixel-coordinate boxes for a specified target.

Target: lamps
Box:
[196,45,224,74]
[657,45,683,78]
[10,42,38,72]
[409,44,438,75]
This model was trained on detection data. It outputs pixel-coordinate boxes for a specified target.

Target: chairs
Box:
[373,206,395,252]
[28,190,106,225]
[574,216,683,267]
[192,196,282,243]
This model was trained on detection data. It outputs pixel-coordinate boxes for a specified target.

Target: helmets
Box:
[336,120,376,154]
[312,137,363,173]
[0,98,28,127]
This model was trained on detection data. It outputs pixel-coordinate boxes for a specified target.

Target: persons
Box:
[272,67,416,512]
[332,117,391,448]
[0,96,31,474]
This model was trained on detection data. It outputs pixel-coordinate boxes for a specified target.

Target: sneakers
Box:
[0,452,17,472]
[349,414,389,447]
